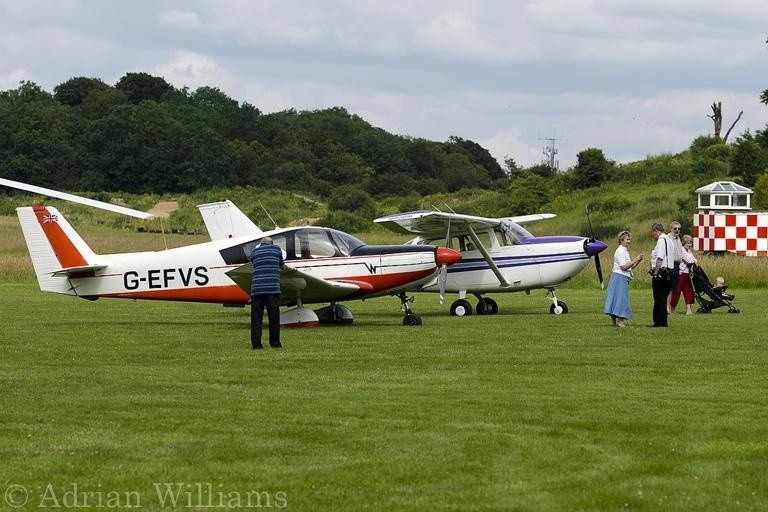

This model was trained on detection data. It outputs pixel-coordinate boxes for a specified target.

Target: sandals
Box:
[610,315,626,327]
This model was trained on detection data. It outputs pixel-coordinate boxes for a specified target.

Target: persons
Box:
[667,221,681,315]
[602,230,644,328]
[647,223,675,326]
[714,274,735,300]
[251,235,285,348]
[669,235,701,315]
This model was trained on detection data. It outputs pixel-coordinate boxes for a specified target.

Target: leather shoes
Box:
[647,322,668,327]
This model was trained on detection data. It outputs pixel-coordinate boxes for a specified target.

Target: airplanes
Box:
[16,190,466,332]
[0,175,156,223]
[365,204,611,318]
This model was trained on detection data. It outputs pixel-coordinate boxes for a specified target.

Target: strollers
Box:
[687,260,744,316]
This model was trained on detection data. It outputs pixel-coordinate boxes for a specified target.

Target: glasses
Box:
[673,227,681,230]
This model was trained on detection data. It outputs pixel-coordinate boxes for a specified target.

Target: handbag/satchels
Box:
[650,266,671,282]
